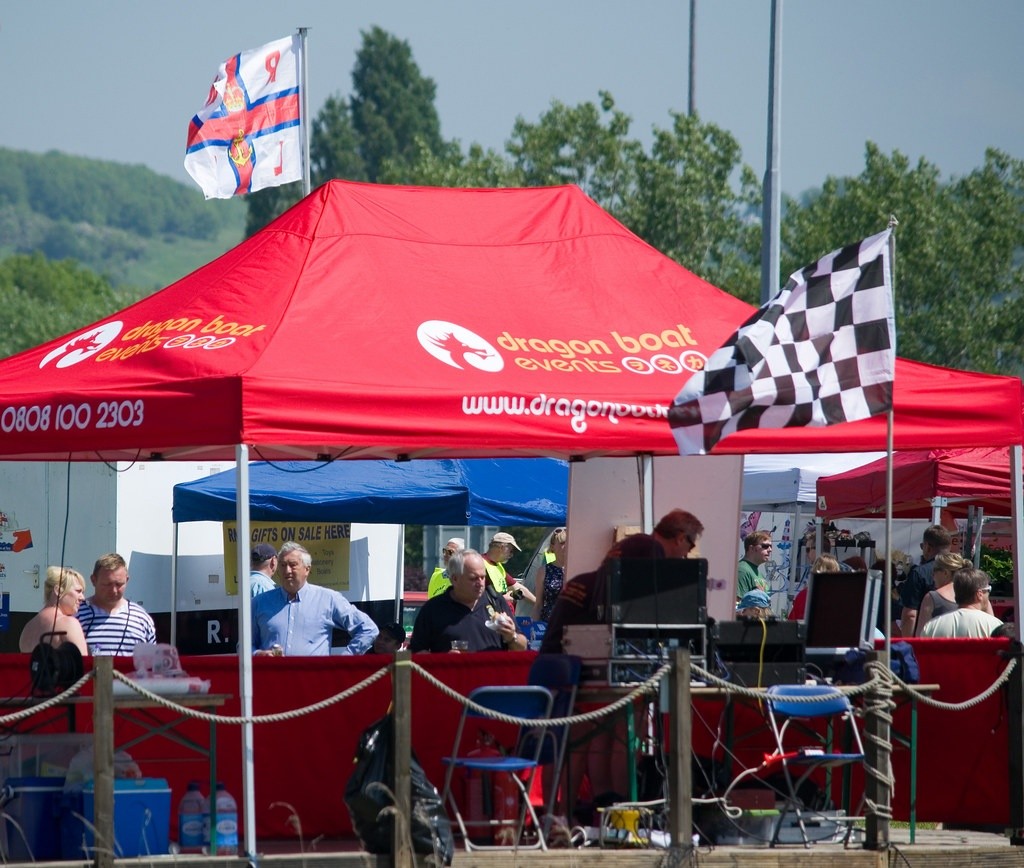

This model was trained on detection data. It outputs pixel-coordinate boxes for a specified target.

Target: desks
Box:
[579,684,940,842]
[0,692,231,856]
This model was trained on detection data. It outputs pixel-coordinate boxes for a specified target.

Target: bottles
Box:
[203,784,239,855]
[177,780,207,851]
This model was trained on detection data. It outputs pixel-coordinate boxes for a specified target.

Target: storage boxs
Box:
[0,777,172,863]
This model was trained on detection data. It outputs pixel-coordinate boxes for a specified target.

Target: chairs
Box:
[766,685,867,849]
[438,683,553,854]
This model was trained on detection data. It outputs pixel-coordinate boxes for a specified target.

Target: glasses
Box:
[553,527,565,540]
[933,566,942,572]
[974,585,992,594]
[805,546,815,553]
[919,542,932,550]
[752,543,773,549]
[444,547,454,557]
[679,527,696,551]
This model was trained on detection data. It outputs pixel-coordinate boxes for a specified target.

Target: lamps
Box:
[191,590,201,605]
[130,595,143,605]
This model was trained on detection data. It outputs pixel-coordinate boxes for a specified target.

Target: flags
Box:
[666,225,895,457]
[186,34,303,199]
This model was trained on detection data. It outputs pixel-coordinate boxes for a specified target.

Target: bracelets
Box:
[508,633,518,644]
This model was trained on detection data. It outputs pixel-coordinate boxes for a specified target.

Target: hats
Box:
[492,532,524,554]
[250,544,281,565]
[737,589,772,611]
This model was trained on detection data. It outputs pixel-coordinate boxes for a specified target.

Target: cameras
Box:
[510,589,525,601]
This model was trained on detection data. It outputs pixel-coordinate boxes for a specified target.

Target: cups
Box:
[451,640,468,653]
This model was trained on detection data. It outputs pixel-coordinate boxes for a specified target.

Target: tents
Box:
[742,450,967,614]
[0,181,1024,868]
[169,459,572,658]
[814,446,1024,571]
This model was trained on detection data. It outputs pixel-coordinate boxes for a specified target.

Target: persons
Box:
[733,524,1017,638]
[20,566,88,655]
[534,528,567,621]
[479,532,535,616]
[236,541,379,656]
[251,543,278,596]
[427,538,465,599]
[595,508,704,623]
[74,553,156,655]
[540,571,599,656]
[410,549,529,654]
[364,623,406,654]
